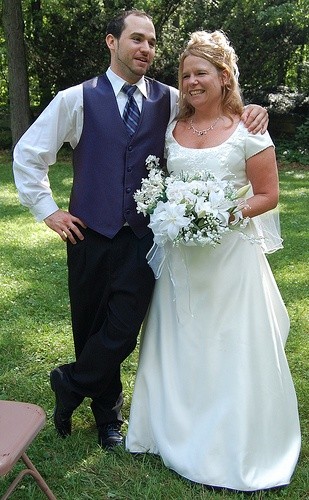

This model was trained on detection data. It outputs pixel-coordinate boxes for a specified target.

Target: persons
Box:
[122,30,301,491]
[13,11,269,451]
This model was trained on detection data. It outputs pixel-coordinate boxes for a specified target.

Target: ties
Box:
[118,83,141,132]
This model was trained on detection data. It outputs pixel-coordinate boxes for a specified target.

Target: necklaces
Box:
[186,113,222,137]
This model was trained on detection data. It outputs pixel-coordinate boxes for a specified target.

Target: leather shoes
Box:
[50,363,83,438]
[97,425,126,453]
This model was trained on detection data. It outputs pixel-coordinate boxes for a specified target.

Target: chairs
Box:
[0,400,57,500]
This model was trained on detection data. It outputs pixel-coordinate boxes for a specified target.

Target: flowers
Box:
[132,154,269,324]
